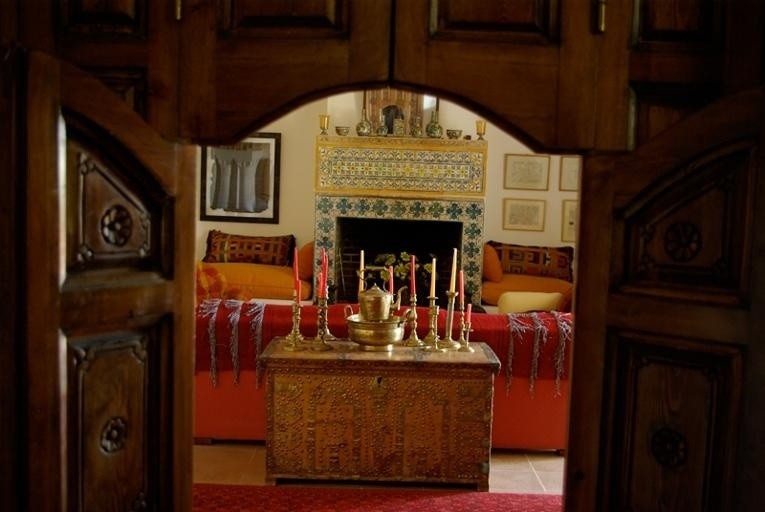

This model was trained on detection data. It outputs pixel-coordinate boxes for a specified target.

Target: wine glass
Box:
[476,121,486,140]
[320,115,329,135]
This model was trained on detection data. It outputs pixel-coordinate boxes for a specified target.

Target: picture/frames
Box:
[559,156,580,192]
[200,131,282,225]
[561,199,578,243]
[504,153,551,192]
[502,198,546,232]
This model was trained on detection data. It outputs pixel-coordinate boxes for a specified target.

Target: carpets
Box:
[192,481,565,511]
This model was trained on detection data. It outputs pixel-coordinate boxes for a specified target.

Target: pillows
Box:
[202,229,295,267]
[487,241,574,281]
[483,246,502,281]
[499,291,562,315]
[299,242,314,281]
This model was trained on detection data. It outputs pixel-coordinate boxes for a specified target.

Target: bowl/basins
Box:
[335,127,351,136]
[447,130,462,140]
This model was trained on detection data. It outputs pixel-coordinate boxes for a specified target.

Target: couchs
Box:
[481,273,573,315]
[194,300,573,459]
[197,262,313,306]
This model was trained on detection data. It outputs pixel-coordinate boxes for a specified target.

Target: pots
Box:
[344,305,412,352]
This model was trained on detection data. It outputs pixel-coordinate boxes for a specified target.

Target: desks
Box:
[260,335,501,493]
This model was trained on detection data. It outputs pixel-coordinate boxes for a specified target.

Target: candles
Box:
[410,254,415,295]
[459,270,464,304]
[389,265,393,294]
[294,247,299,288]
[466,303,471,324]
[430,257,436,297]
[297,280,301,304]
[359,250,364,292]
[321,255,329,298]
[321,248,325,276]
[449,248,458,292]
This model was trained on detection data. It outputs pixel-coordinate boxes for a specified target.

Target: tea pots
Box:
[357,265,407,322]
